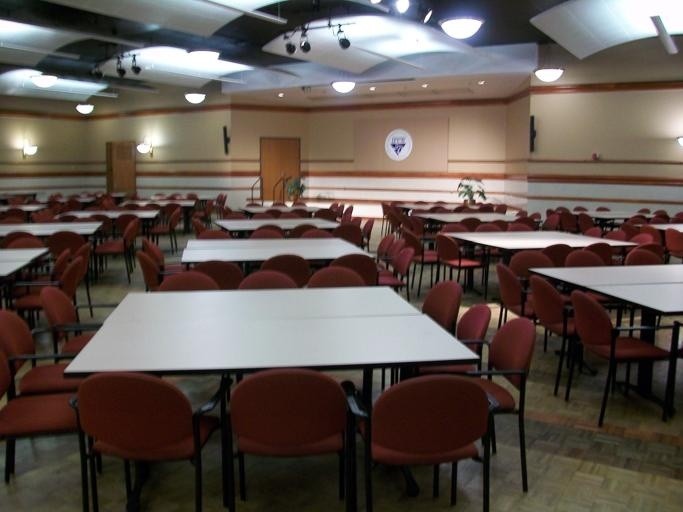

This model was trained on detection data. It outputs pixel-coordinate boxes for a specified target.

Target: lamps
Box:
[438,15,486,40]
[116,53,141,77]
[279,24,353,54]
